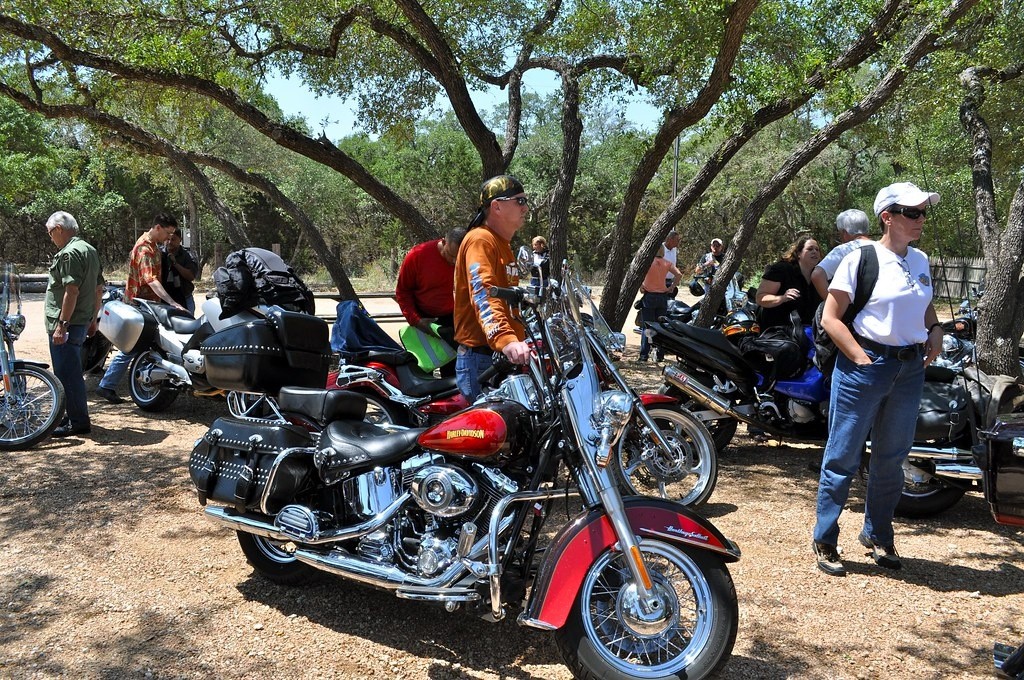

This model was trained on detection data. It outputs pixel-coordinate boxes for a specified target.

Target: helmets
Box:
[722,307,761,339]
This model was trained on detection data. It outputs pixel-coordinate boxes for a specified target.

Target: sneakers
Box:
[858,529,900,569]
[812,540,844,576]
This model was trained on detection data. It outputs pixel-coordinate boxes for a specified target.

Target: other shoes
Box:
[655,358,665,363]
[635,358,648,364]
[96,387,123,404]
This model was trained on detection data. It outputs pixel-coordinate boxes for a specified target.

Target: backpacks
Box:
[813,246,879,372]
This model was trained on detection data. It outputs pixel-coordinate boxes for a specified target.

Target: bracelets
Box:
[171,260,177,266]
[927,322,942,335]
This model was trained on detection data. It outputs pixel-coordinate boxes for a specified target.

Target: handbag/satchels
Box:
[739,311,806,371]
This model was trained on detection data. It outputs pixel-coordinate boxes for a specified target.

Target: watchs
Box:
[59,320,70,328]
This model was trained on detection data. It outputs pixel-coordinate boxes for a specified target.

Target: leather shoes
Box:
[52,421,91,436]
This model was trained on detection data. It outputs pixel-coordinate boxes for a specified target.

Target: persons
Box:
[812,209,876,475]
[161,229,199,316]
[755,235,822,431]
[812,182,942,576]
[661,231,680,288]
[43,210,103,436]
[960,296,969,308]
[396,227,467,379]
[96,211,190,404]
[454,176,538,548]
[517,236,550,287]
[695,238,725,297]
[631,244,682,364]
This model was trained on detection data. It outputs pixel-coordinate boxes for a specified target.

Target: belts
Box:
[464,344,494,357]
[852,334,924,363]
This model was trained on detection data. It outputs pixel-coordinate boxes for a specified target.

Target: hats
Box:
[478,175,524,211]
[873,182,940,217]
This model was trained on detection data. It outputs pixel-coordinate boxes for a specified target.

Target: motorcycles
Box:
[295,260,717,514]
[849,285,1023,531]
[97,276,271,413]
[82,274,127,376]
[188,246,745,680]
[0,260,66,453]
[628,276,838,461]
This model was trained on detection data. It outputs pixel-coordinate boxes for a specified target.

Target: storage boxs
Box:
[98,299,156,356]
[199,306,333,391]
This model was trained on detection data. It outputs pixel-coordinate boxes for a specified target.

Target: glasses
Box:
[886,208,927,219]
[163,227,173,236]
[495,196,527,207]
[46,227,57,238]
